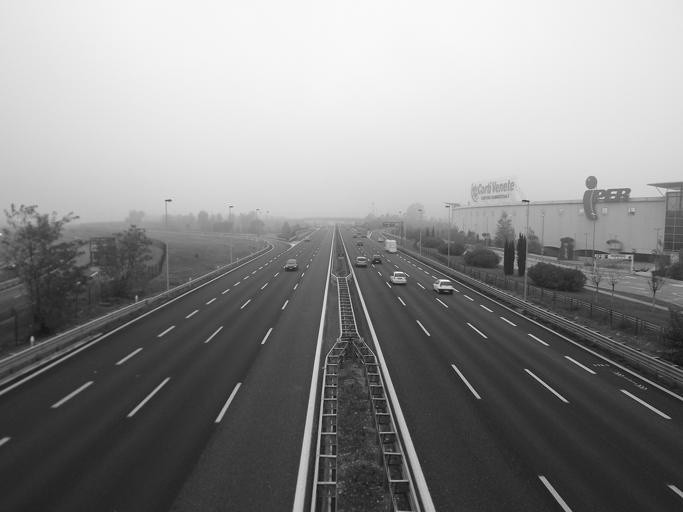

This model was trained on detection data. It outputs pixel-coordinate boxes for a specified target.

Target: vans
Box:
[355,256,368,267]
[432,279,455,294]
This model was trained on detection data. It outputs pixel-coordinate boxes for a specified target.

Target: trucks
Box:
[383,239,397,253]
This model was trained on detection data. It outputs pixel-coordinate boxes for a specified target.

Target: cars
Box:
[377,237,384,242]
[345,225,369,247]
[389,271,407,285]
[282,258,298,272]
[370,254,382,264]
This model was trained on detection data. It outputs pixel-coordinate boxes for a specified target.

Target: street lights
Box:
[443,205,451,268]
[163,198,173,290]
[519,199,530,300]
[590,208,599,266]
[253,208,260,250]
[539,211,544,255]
[227,204,234,264]
[417,208,423,256]
